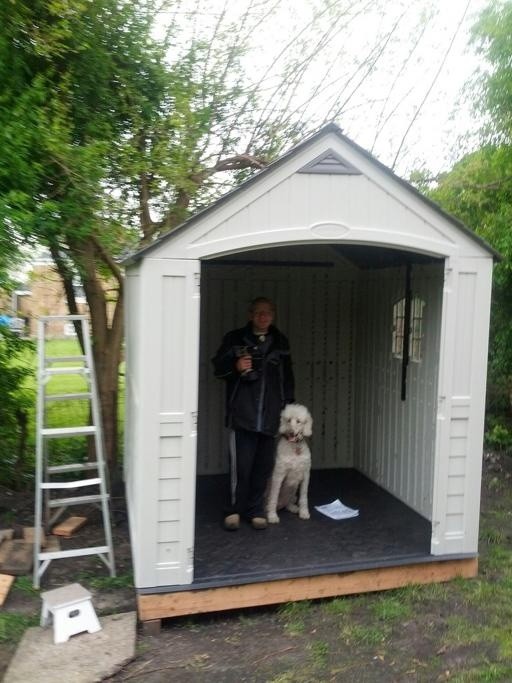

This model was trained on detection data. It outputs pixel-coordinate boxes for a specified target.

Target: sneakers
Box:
[224,514,241,529]
[252,517,268,530]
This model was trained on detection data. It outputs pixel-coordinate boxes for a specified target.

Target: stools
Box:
[39,582,102,644]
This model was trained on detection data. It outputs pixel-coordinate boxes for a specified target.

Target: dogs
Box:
[263,403,314,524]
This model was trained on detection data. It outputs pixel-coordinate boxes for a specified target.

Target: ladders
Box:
[32,315,116,589]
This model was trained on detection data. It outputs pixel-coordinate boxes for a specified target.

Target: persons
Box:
[211,297,295,531]
